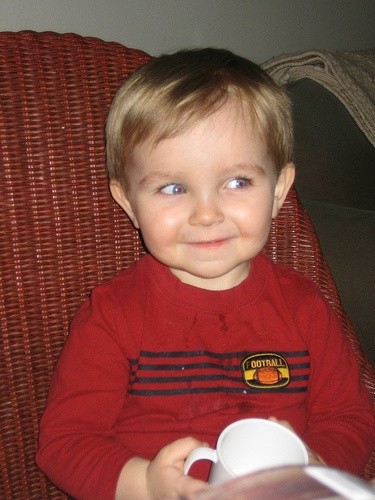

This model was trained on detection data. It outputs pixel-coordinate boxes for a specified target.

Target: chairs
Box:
[0,30,375,500]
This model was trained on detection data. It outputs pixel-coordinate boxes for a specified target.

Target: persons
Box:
[37,47,374,500]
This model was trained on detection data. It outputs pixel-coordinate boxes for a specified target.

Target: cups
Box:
[185,418,308,487]
